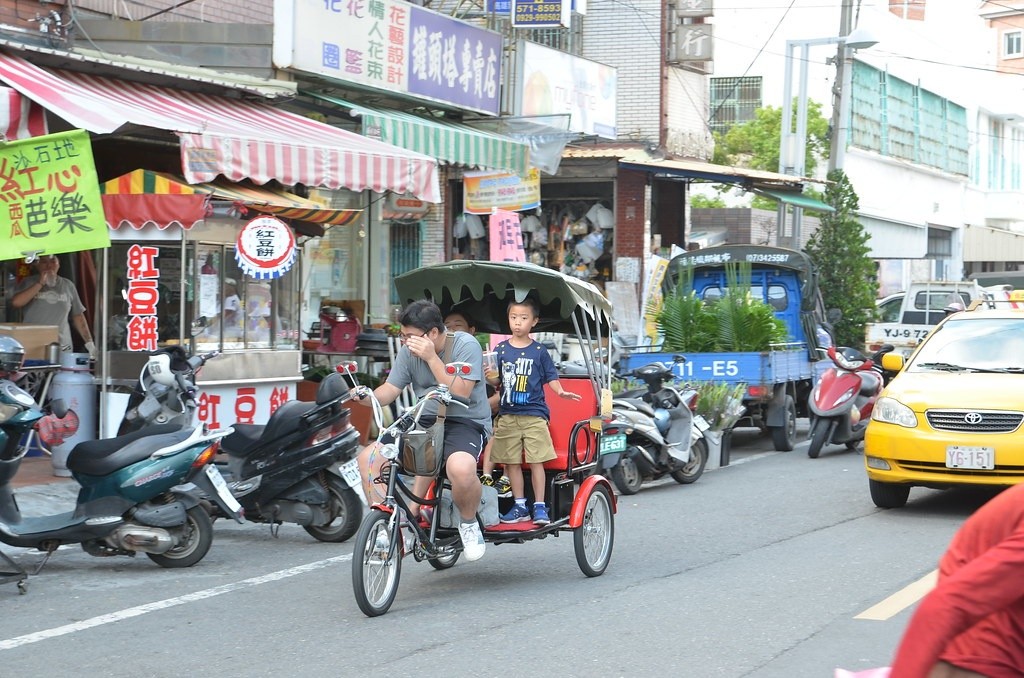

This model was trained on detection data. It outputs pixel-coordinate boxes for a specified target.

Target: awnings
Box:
[298,90,529,174]
[0,51,443,207]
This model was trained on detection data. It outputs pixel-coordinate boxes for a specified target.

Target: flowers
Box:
[609,377,750,432]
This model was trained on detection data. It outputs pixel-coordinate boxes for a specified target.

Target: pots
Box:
[321,304,341,313]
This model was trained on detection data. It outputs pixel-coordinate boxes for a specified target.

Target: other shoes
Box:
[420,507,433,523]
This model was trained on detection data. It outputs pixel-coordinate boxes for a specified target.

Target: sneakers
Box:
[458,521,485,561]
[501,503,531,523]
[492,476,513,498]
[479,475,494,487]
[365,530,391,556]
[532,504,551,524]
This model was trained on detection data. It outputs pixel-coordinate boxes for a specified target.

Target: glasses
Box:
[398,330,429,341]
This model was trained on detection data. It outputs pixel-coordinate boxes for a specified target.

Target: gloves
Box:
[85,342,95,353]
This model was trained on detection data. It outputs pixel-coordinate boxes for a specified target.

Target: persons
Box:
[11,255,95,360]
[351,300,493,562]
[481,291,582,523]
[397,311,511,526]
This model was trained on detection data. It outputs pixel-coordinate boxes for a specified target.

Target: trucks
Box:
[617,243,843,454]
[850,269,1024,382]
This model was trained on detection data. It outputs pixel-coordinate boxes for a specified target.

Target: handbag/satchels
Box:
[403,418,444,476]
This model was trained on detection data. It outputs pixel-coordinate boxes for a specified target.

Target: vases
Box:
[703,429,733,469]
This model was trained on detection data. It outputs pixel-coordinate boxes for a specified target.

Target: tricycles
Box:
[336,258,619,619]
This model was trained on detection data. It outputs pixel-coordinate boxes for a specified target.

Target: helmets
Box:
[944,303,965,313]
[149,345,190,386]
[654,408,671,431]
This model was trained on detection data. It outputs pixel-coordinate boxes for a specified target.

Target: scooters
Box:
[111,315,374,544]
[0,365,247,594]
[595,353,719,494]
[805,344,898,458]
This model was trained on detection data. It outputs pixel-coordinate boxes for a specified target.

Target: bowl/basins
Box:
[303,340,324,349]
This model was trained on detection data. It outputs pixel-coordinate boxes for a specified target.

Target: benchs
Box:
[475,378,598,474]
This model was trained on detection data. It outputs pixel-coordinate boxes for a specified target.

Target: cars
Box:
[862,306,1024,510]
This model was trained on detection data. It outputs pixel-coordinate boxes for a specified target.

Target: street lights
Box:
[776,27,881,248]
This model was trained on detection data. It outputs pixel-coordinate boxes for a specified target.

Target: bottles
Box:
[46,270,56,287]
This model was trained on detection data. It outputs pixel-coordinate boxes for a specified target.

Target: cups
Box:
[483,352,499,380]
[45,344,60,364]
[653,234,662,247]
